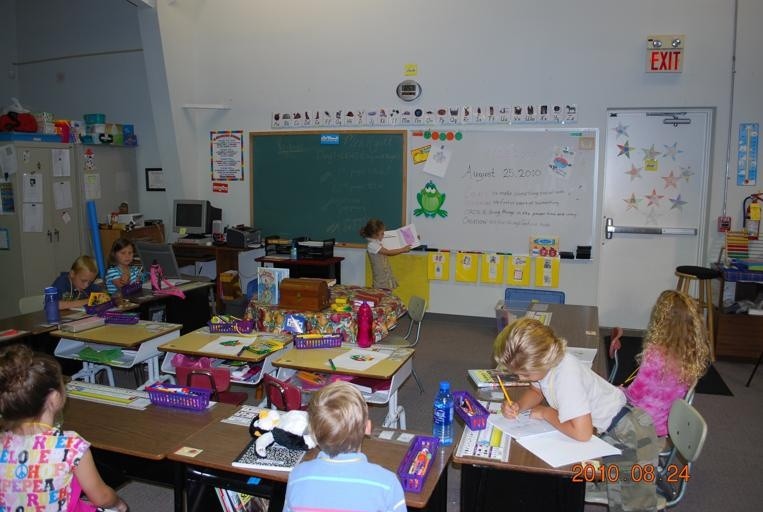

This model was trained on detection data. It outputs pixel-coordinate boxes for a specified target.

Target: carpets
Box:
[603,335,734,397]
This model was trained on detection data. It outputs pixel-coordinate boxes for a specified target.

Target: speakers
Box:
[212,219,224,245]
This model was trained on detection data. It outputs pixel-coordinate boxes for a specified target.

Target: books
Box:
[468,368,531,389]
[322,344,393,396]
[214,485,270,512]
[231,434,304,473]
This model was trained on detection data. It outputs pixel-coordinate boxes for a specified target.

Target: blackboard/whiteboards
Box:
[249,130,407,249]
[409,127,600,262]
[601,106,716,235]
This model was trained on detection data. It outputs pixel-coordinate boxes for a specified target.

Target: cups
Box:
[43,123,57,136]
[290,248,297,259]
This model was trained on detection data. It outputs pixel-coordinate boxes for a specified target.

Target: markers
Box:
[157,387,190,393]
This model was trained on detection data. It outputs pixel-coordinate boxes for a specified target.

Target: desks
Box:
[712,265,762,362]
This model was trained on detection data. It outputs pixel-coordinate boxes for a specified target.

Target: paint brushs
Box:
[329,359,336,371]
[408,448,432,488]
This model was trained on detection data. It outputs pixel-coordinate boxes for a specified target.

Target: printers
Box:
[227,224,265,249]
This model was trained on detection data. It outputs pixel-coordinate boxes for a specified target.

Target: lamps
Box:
[648,35,685,48]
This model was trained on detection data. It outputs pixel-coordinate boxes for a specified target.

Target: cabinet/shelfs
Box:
[71,144,138,262]
[0,139,83,319]
[94,223,164,269]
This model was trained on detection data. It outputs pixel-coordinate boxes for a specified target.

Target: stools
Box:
[676,265,717,348]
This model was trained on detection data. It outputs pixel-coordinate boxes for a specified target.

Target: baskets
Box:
[293,333,341,349]
[446,394,490,431]
[398,436,440,493]
[147,383,210,411]
[206,320,253,333]
[83,281,141,324]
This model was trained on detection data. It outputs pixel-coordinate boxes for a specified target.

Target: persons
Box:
[0,343,127,511]
[487,409,622,469]
[360,218,411,292]
[284,379,406,511]
[43,253,103,309]
[617,290,708,434]
[493,318,660,512]
[104,237,145,296]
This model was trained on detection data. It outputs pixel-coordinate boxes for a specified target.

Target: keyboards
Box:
[174,239,211,246]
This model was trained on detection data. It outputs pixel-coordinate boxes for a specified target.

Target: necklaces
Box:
[21,422,52,429]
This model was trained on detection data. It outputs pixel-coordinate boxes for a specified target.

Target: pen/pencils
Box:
[496,374,522,423]
[495,252,513,256]
[464,398,474,415]
[458,251,484,255]
[80,287,90,298]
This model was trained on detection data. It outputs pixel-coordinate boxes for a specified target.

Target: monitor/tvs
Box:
[171,200,222,239]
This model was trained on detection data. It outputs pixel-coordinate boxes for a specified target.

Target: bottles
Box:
[532,246,556,257]
[357,299,373,348]
[433,382,454,447]
[44,287,60,323]
[150,259,161,292]
[110,212,118,224]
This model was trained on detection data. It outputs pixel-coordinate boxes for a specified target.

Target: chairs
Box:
[606,325,622,385]
[585,400,707,512]
[655,374,698,457]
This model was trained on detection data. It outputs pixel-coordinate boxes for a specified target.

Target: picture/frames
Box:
[145,168,166,191]
